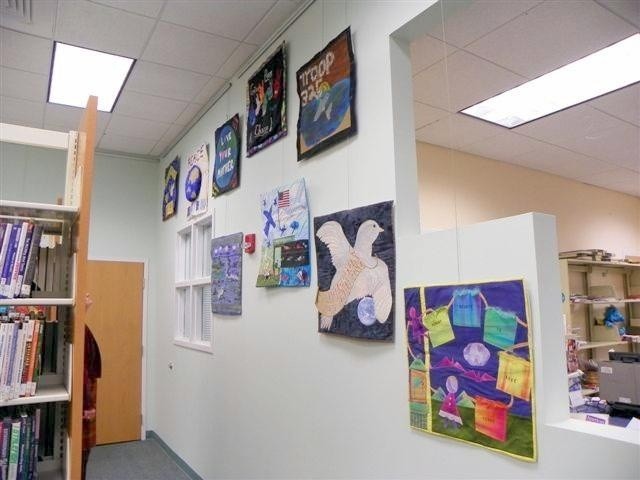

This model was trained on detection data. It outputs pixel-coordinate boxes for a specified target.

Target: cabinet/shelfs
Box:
[559,258,640,399]
[0,94,97,479]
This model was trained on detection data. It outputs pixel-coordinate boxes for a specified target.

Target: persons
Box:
[80,323,101,480]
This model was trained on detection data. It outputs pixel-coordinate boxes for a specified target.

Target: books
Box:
[558,246,640,264]
[0,220,47,480]
